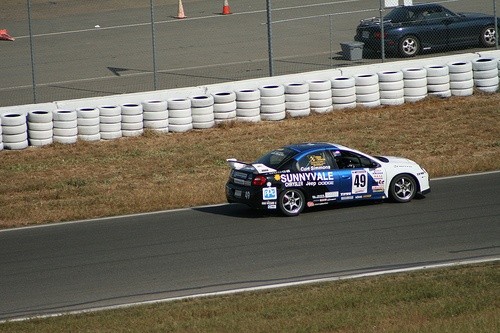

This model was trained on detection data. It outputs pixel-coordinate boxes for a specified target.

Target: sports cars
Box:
[355,3,500,56]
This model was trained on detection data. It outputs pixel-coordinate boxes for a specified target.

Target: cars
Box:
[224,141,431,216]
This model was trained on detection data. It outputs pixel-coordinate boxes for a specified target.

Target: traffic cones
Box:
[221,0,232,15]
[0,29,16,41]
[172,0,188,19]
